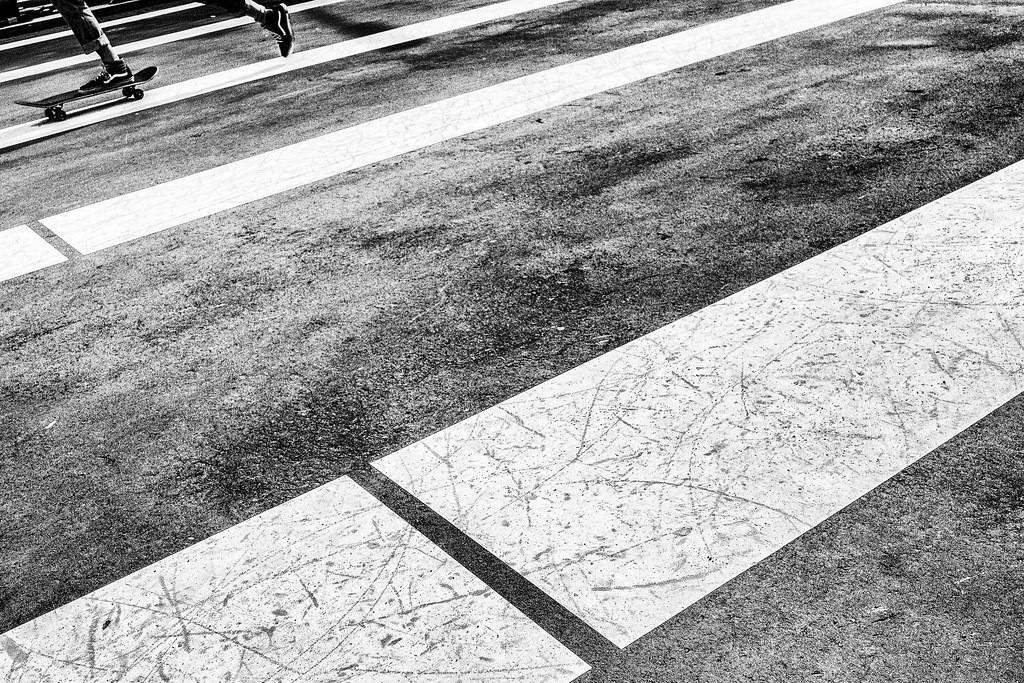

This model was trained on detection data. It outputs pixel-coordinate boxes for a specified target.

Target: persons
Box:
[51,0,295,94]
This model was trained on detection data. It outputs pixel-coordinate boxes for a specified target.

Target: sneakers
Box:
[78,57,135,95]
[260,3,294,58]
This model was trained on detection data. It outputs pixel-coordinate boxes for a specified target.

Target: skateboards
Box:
[14,66,159,122]
